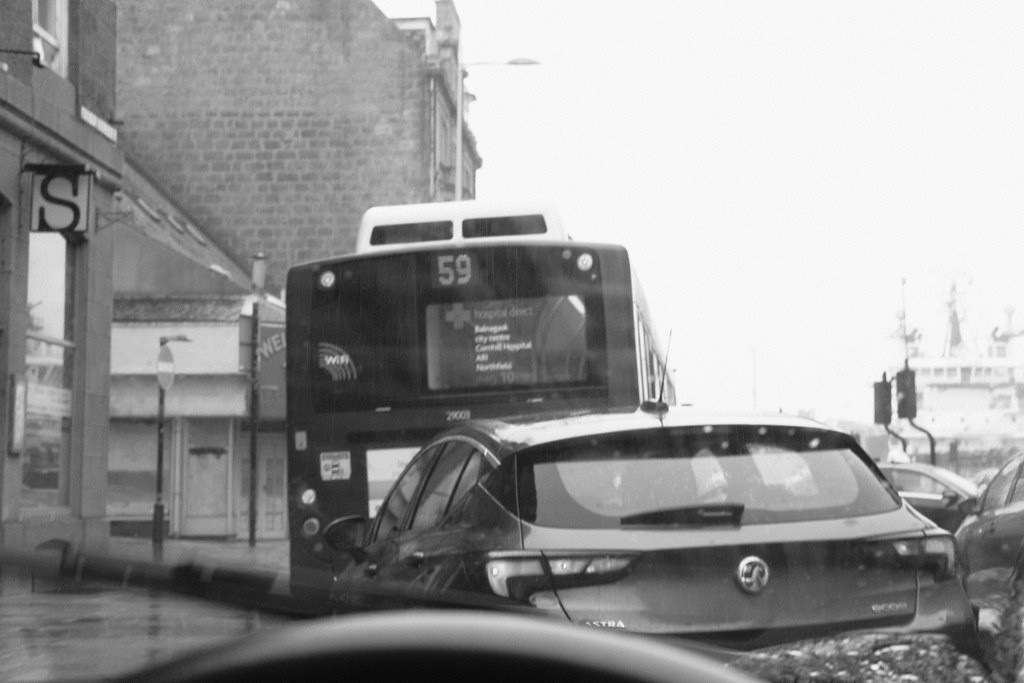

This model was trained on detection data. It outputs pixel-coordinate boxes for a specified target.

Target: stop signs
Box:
[157,345,175,389]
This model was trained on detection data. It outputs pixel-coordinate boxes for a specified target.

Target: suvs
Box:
[323,398,980,675]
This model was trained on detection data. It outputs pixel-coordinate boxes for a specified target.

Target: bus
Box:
[287,200,678,617]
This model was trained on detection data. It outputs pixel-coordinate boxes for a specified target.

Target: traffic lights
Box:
[896,369,916,418]
[875,382,891,424]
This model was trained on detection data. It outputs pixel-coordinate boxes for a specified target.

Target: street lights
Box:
[453,57,543,205]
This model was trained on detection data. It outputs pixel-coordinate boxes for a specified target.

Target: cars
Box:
[950,453,1024,613]
[875,458,980,531]
